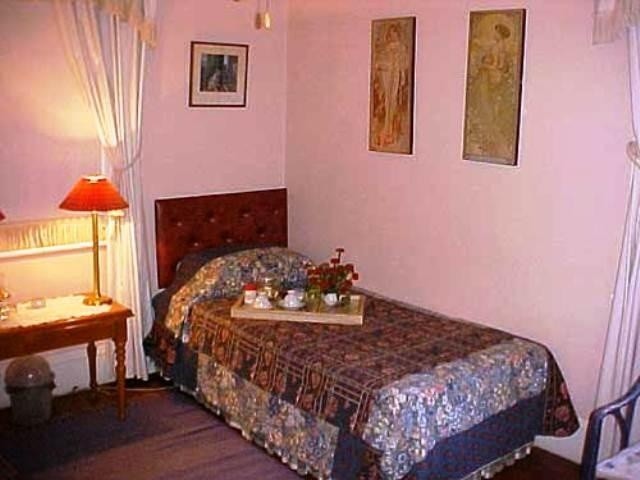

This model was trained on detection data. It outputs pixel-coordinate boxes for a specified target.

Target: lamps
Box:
[265,0,271,30]
[59,176,129,307]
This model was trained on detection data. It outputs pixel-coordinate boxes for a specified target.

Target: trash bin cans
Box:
[5,356,56,424]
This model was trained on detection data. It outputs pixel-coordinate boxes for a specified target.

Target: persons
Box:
[208,63,223,90]
[472,24,511,73]
[376,24,409,145]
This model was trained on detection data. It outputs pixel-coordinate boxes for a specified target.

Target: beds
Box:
[144,187,580,480]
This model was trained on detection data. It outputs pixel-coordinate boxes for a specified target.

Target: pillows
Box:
[176,243,315,297]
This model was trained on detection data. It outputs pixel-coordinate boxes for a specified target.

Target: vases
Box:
[319,289,346,306]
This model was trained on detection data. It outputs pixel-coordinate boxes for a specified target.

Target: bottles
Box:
[239,270,322,312]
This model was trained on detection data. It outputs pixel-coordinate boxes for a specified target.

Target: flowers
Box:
[302,248,359,295]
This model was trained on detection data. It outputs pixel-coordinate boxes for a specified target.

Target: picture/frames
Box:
[189,41,249,107]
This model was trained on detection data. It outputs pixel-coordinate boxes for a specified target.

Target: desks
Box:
[0,293,134,425]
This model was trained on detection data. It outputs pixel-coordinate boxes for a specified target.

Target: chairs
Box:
[579,374,640,480]
